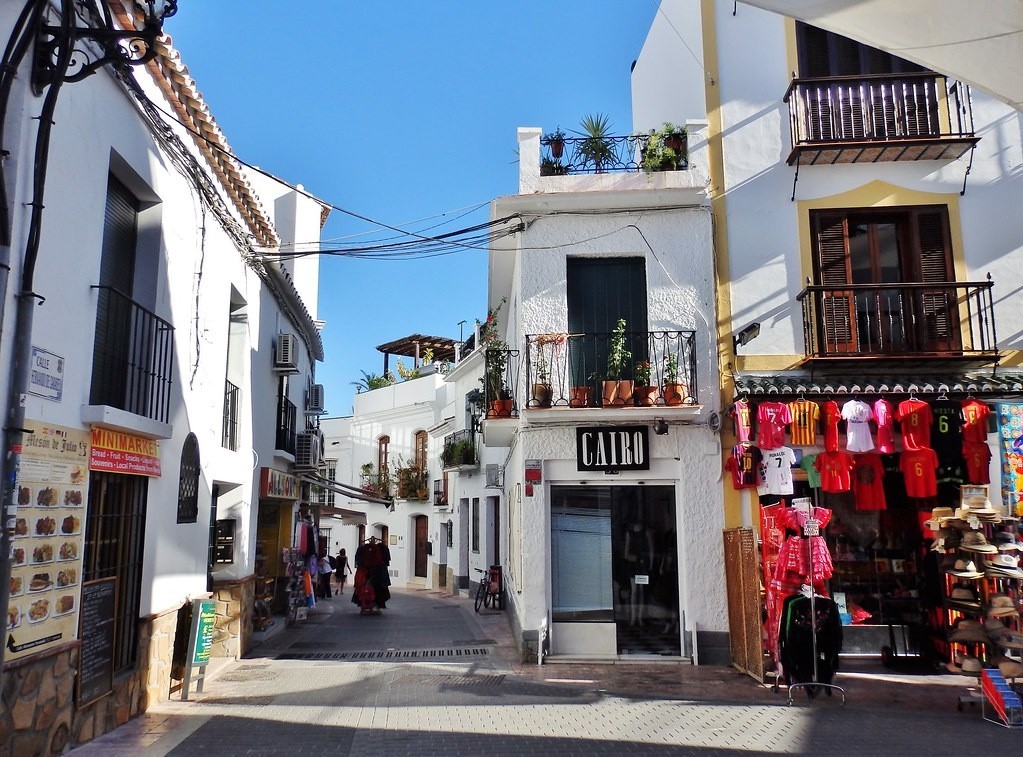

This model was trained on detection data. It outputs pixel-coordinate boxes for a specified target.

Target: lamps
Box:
[734,322,760,355]
[653,417,670,436]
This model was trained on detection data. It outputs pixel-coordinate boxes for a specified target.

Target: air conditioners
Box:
[309,384,324,409]
[295,433,320,469]
[305,429,325,461]
[276,333,300,368]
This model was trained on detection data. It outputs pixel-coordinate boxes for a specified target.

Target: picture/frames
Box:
[960,484,991,509]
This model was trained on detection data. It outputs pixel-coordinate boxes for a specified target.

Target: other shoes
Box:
[325,597,332,600]
[335,590,339,595]
[374,609,383,616]
[360,608,370,616]
[381,603,386,608]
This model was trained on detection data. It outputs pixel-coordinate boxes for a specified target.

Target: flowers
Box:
[476,295,682,410]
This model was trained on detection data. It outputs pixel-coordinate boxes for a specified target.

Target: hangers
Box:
[910,391,918,400]
[742,441,750,444]
[853,394,860,401]
[936,391,949,400]
[798,583,819,599]
[967,392,975,399]
[809,446,820,455]
[797,392,805,402]
[741,393,748,402]
[879,393,884,399]
[823,394,830,401]
[769,394,778,403]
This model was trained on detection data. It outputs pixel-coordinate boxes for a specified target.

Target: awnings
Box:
[313,502,367,526]
[308,474,395,512]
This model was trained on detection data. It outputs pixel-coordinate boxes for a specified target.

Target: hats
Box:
[925,492,1023,680]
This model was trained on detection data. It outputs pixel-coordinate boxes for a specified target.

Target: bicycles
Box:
[474,568,492,613]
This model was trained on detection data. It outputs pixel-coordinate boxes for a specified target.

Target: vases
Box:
[662,383,690,406]
[601,380,635,408]
[570,386,595,408]
[531,383,553,406]
[488,401,496,419]
[634,385,659,404]
[495,400,513,417]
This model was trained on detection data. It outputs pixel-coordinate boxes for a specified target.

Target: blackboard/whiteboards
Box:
[75,576,119,710]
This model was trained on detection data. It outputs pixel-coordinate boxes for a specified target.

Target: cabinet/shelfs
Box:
[255,577,274,627]
[832,548,921,624]
[935,520,1019,678]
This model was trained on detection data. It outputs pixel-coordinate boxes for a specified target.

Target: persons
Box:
[335,549,352,596]
[624,522,654,626]
[319,551,332,600]
[659,526,680,634]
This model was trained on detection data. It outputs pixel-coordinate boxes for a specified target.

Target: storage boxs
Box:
[982,669,1023,726]
